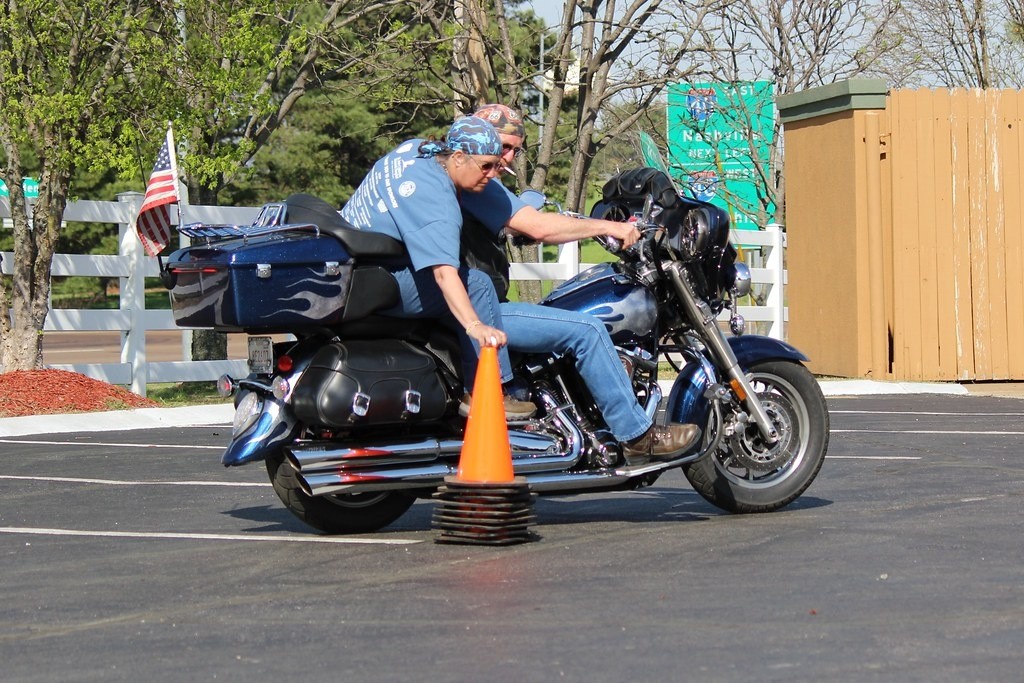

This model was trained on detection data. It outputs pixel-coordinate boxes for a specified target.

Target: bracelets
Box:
[465,319,483,335]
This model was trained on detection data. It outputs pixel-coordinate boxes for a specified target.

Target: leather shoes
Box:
[621,423,703,463]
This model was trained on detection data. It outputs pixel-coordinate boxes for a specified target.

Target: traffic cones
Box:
[429,336,541,545]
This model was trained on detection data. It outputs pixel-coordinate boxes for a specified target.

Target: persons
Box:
[456,104,702,464]
[340,116,536,420]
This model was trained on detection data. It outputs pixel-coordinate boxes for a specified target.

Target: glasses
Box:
[468,154,502,171]
[501,143,520,154]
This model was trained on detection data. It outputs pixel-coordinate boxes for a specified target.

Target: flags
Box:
[136,126,180,259]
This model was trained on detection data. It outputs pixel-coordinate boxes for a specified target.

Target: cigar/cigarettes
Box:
[504,166,517,176]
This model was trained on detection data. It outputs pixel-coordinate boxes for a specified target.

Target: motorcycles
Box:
[131,133,832,535]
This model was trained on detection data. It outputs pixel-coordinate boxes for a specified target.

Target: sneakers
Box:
[458,388,538,422]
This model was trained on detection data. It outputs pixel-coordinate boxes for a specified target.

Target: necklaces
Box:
[440,155,449,175]
